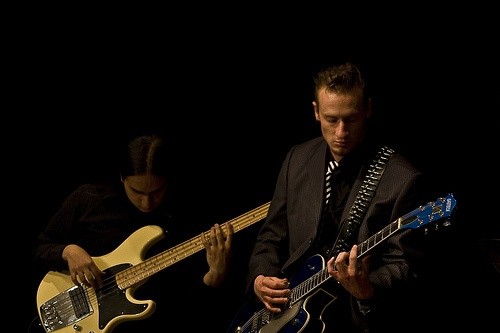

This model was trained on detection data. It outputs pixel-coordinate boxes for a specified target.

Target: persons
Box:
[33,133,233,333]
[247,61,433,333]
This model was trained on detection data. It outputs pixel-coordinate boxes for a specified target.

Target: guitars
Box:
[36,201,271,333]
[230,193,457,333]
